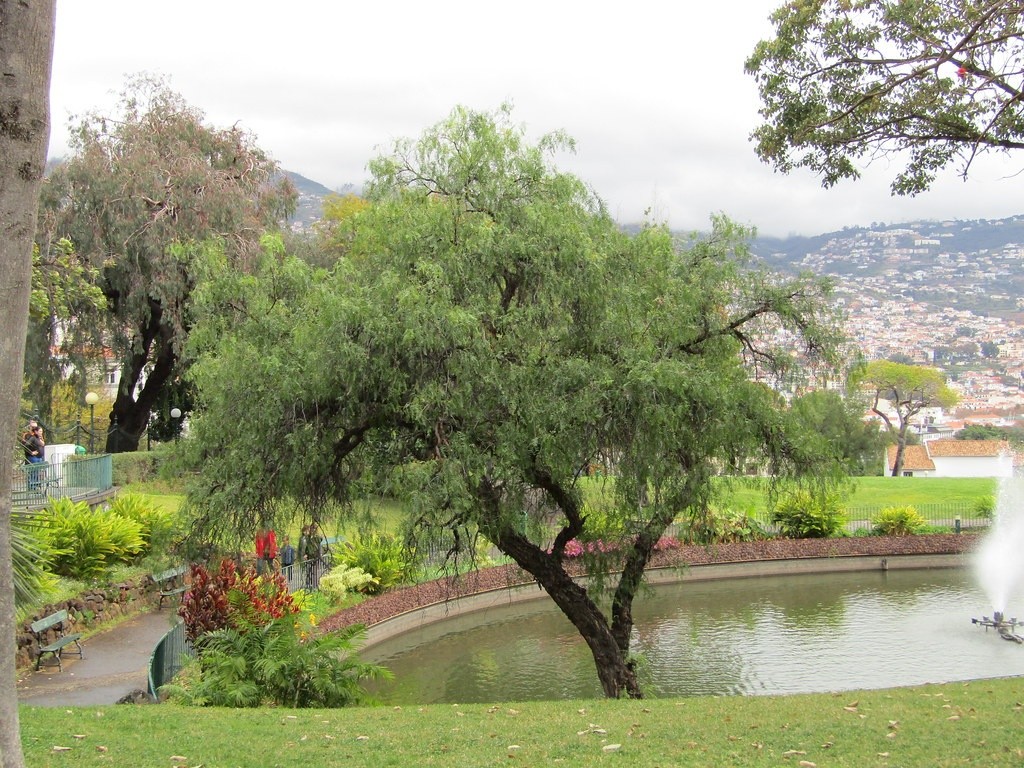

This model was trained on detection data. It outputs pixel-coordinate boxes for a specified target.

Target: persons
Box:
[22,420,45,494]
[256,524,324,588]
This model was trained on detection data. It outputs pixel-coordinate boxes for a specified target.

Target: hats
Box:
[30,420,37,427]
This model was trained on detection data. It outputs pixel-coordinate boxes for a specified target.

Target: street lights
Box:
[85,392,99,454]
[171,408,182,447]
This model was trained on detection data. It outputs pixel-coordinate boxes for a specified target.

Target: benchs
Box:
[31,609,83,672]
[22,461,58,498]
[152,562,192,614]
